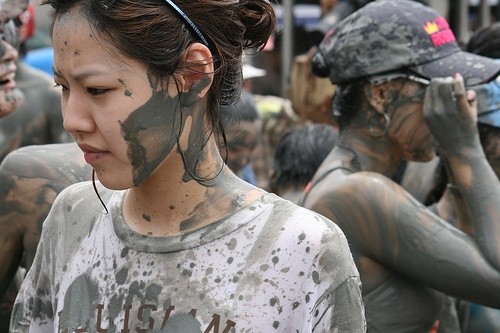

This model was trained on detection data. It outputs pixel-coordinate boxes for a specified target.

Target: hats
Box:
[312,0,500,87]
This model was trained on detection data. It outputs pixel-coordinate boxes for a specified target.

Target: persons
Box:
[302,0,500,333]
[0,0,96,333]
[436,22,500,333]
[219,54,338,205]
[9,0,367,333]
